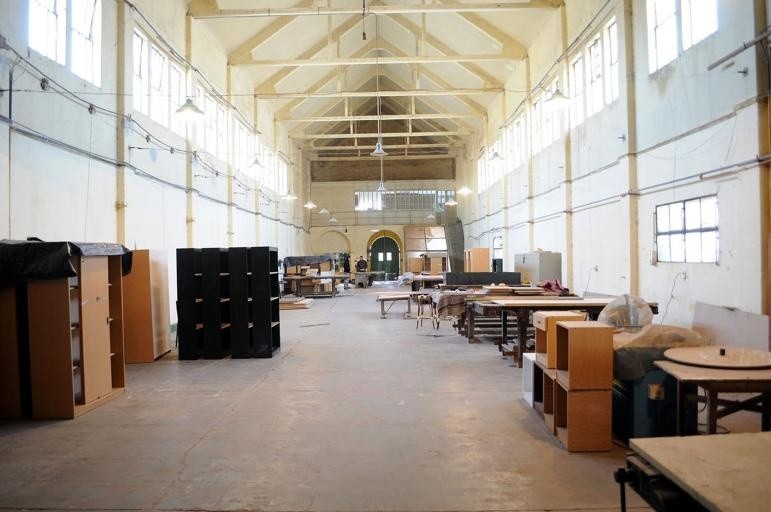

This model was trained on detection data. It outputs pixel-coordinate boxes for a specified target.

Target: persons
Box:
[344,256,352,289]
[356,255,368,272]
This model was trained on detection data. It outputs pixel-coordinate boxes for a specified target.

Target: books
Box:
[478,282,549,298]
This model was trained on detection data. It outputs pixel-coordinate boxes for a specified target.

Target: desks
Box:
[283,275,350,299]
[652,359,770,436]
[413,283,615,367]
[354,272,372,288]
[613,432,770,511]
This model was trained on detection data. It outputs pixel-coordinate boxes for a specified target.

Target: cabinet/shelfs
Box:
[122,248,172,364]
[0,239,126,420]
[283,255,333,294]
[176,245,280,359]
[522,310,613,453]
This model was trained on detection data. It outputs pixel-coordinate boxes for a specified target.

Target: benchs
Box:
[376,294,410,319]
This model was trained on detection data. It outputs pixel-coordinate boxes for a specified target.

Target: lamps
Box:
[427,152,473,219]
[377,156,386,191]
[370,97,390,156]
[176,67,338,225]
